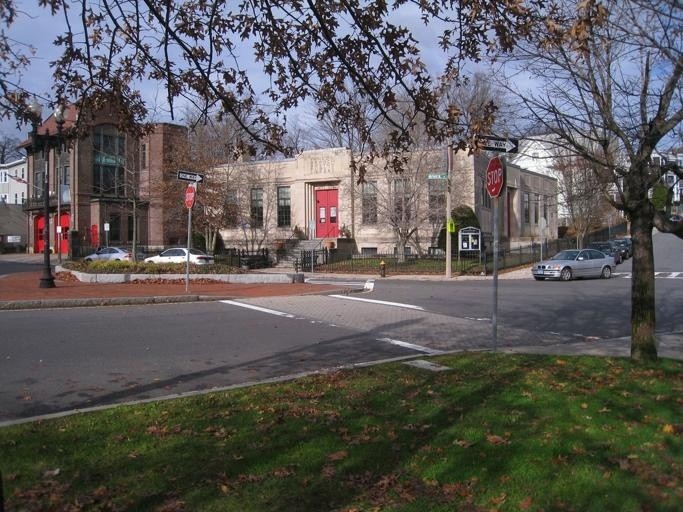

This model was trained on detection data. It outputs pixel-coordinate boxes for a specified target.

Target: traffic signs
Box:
[473,131,519,155]
[177,170,205,183]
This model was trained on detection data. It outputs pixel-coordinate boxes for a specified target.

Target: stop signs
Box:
[185,185,196,207]
[483,155,505,197]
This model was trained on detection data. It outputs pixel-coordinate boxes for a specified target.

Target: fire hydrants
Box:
[379,259,385,277]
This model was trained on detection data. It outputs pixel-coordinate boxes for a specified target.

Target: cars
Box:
[531,248,617,281]
[597,236,632,264]
[75,244,144,265]
[137,246,216,266]
